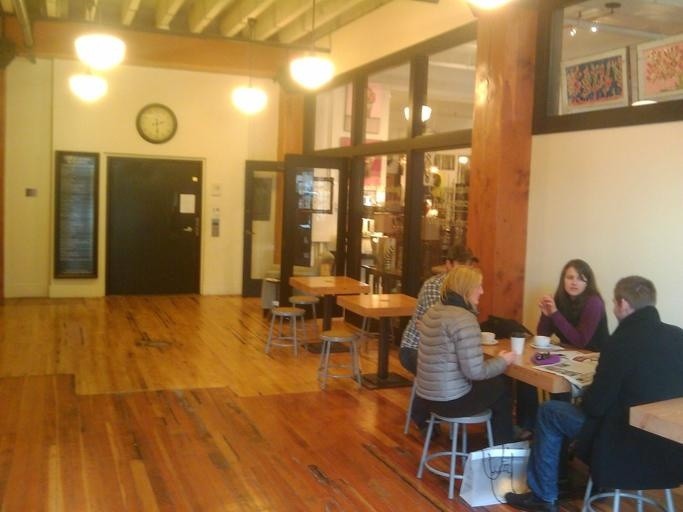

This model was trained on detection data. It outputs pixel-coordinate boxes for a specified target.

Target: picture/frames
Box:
[636,33,683,99]
[297,177,334,214]
[560,47,629,114]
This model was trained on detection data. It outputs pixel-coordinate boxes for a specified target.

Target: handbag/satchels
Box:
[460,438,533,508]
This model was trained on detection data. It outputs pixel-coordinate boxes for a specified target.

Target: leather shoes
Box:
[556,482,608,502]
[505,492,558,512]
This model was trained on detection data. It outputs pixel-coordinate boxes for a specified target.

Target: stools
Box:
[581,465,675,512]
[404,371,453,443]
[416,408,495,500]
[264,295,395,390]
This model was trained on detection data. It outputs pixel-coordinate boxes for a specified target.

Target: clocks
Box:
[137,103,177,144]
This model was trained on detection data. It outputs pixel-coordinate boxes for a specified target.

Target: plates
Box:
[529,345,564,352]
[480,339,499,345]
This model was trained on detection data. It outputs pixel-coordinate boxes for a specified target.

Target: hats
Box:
[442,246,479,263]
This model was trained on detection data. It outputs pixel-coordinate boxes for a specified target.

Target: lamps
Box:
[232,18,267,115]
[288,0,335,89]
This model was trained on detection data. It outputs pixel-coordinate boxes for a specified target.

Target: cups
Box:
[481,329,497,343]
[510,335,526,354]
[534,336,551,347]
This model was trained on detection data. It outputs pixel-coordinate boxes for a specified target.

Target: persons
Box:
[514,259,609,440]
[398,244,479,439]
[504,276,683,512]
[414,264,514,446]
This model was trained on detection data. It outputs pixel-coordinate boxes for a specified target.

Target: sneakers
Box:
[414,422,436,439]
